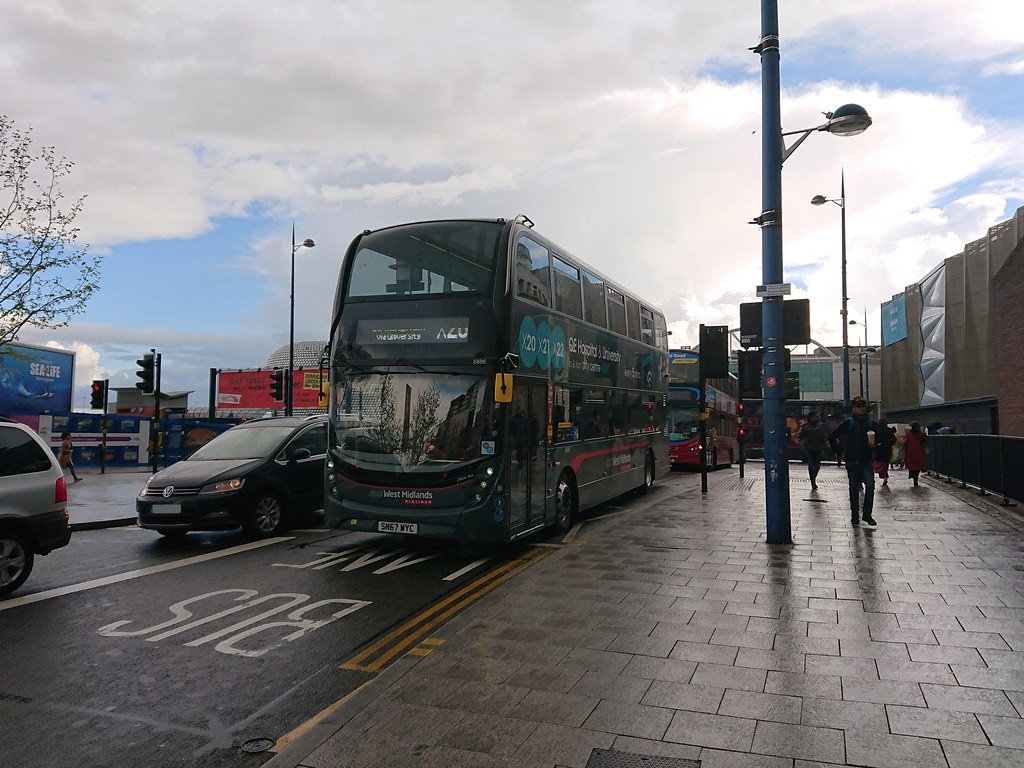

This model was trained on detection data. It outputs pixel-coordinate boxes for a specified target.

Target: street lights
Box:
[760,102,873,541]
[287,239,315,416]
[811,195,850,419]
[849,320,870,401]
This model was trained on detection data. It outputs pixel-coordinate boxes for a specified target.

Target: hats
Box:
[879,418,887,424]
[852,396,867,407]
[909,421,920,427]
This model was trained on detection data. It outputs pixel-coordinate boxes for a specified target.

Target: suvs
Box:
[136,414,329,538]
[0,417,72,598]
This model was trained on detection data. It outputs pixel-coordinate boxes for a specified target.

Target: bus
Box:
[318,213,670,544]
[740,398,882,461]
[669,349,739,472]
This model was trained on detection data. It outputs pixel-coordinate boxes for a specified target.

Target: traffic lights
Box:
[270,369,284,402]
[90,380,104,409]
[136,353,154,396]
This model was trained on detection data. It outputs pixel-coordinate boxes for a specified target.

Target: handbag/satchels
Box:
[59,455,70,468]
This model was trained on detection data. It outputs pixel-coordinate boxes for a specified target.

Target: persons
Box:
[831,438,843,468]
[873,417,897,487]
[57,431,84,484]
[827,396,890,525]
[794,411,831,491]
[904,422,927,488]
[791,418,807,446]
[890,425,905,467]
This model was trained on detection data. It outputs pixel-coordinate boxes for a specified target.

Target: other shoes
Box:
[862,513,877,525]
[75,478,82,483]
[851,510,859,524]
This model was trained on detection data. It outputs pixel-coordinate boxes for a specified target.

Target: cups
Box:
[867,431,875,444]
